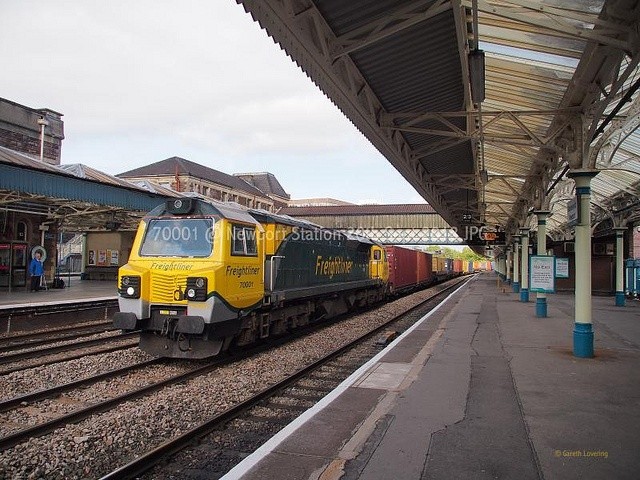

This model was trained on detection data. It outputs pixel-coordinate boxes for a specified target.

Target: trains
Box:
[113,198,492,359]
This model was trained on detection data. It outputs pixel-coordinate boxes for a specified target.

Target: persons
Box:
[29,253,44,293]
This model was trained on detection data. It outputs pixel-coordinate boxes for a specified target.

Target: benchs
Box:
[84,266,119,281]
